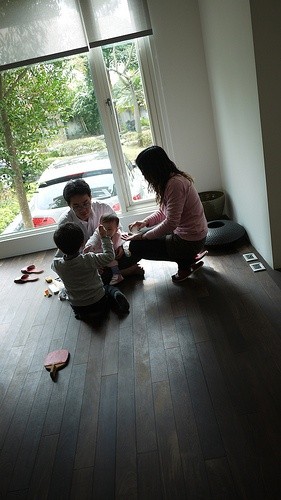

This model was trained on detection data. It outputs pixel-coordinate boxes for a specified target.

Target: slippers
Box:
[190,250,208,266]
[172,261,203,282]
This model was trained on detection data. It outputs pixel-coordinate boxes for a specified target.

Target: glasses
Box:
[70,196,90,211]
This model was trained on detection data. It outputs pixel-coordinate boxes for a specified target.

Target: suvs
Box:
[29,148,143,227]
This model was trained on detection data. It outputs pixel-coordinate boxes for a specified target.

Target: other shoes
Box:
[114,290,130,314]
[110,273,124,285]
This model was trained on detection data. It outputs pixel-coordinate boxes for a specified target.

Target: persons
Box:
[55,146,209,283]
[50,222,130,319]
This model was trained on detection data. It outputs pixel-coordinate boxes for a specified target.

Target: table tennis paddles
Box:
[43,349,69,377]
